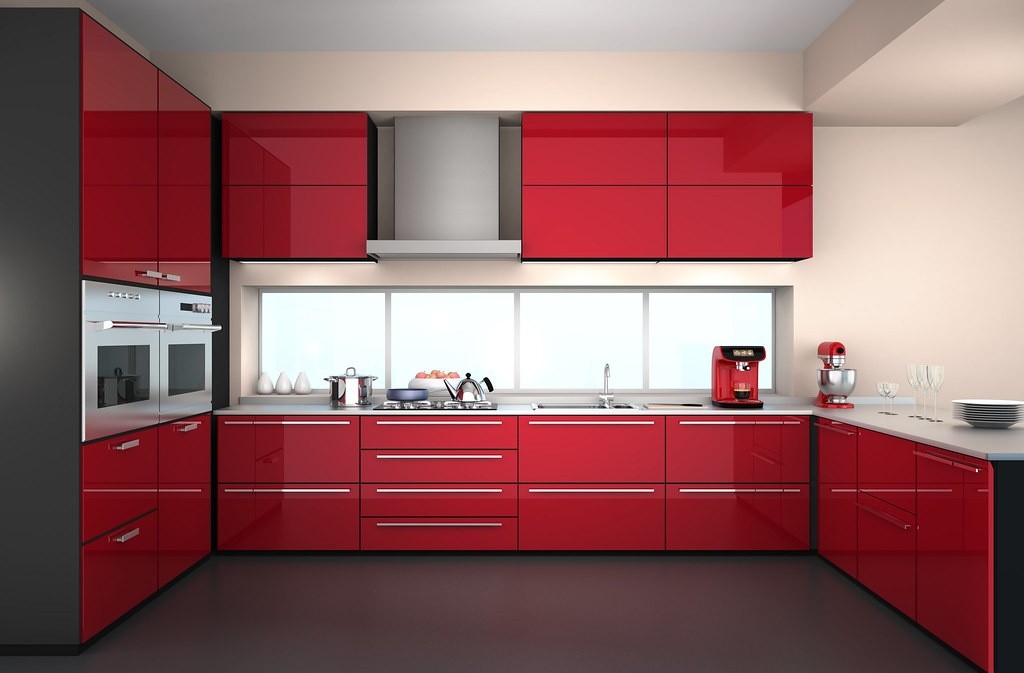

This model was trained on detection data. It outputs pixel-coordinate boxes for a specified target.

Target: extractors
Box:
[363,112,523,264]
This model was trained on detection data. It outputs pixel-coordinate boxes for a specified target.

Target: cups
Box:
[733,382,751,402]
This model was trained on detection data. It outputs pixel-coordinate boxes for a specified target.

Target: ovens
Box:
[77,278,222,443]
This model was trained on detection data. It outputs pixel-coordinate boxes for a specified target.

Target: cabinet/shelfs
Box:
[81,427,158,651]
[360,415,518,550]
[666,415,810,550]
[217,416,360,550]
[81,13,212,293]
[521,113,813,261]
[158,413,211,593]
[518,415,666,551]
[813,417,994,673]
[221,111,378,264]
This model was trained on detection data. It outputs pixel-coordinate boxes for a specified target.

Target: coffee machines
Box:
[710,346,766,407]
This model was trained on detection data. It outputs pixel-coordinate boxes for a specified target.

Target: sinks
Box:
[613,405,633,409]
[537,405,607,408]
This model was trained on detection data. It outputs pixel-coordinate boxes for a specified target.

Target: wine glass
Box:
[915,365,933,420]
[925,366,946,423]
[883,382,899,414]
[876,382,889,413]
[905,364,923,418]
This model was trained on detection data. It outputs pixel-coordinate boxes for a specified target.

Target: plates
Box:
[950,398,1024,429]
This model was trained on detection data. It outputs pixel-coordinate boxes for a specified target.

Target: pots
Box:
[385,388,430,401]
[323,367,378,408]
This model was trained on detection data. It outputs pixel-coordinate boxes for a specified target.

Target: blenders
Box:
[815,341,858,408]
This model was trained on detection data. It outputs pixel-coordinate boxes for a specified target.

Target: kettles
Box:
[443,373,494,403]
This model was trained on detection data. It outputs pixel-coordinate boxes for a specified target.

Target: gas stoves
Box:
[372,400,498,410]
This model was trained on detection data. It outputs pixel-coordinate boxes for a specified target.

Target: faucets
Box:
[736,362,751,372]
[599,364,614,404]
